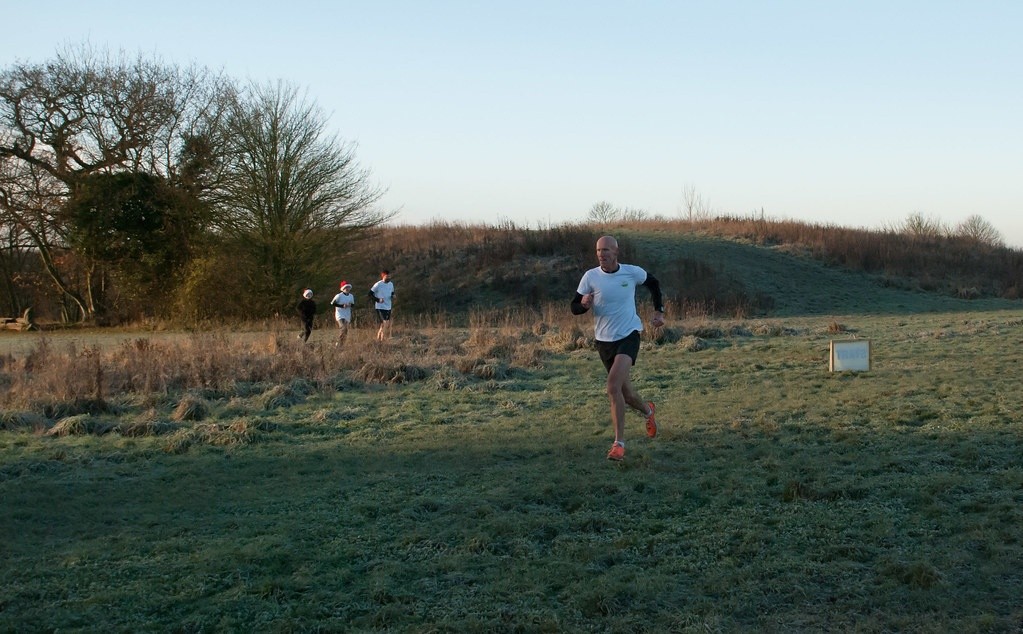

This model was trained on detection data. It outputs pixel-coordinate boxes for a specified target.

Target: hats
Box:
[339,280,352,292]
[301,287,314,298]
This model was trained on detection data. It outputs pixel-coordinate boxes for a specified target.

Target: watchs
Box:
[659,307,664,313]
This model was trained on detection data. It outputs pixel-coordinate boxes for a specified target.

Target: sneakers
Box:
[644,401,658,439]
[607,442,624,461]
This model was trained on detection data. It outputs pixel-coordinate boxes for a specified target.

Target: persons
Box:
[368,271,397,341]
[571,236,665,461]
[331,280,355,348]
[297,289,317,343]
